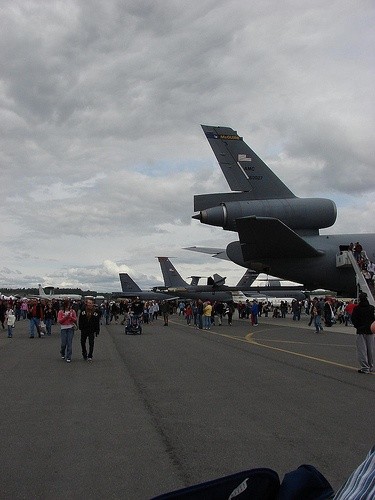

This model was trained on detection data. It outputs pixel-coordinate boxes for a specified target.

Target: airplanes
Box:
[111,124,375,307]
[26,283,84,302]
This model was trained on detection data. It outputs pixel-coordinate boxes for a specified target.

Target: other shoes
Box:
[30,336,34,338]
[358,370,369,373]
[88,358,92,361]
[67,359,71,363]
[62,356,65,359]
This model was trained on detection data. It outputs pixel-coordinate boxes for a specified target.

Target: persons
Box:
[352,292,375,374]
[0,294,375,339]
[78,298,100,361]
[57,301,77,362]
[348,241,375,288]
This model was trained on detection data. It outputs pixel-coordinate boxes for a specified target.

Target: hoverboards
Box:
[125,313,142,336]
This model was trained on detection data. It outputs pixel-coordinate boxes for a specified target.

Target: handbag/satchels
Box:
[71,309,77,330]
[39,320,47,335]
